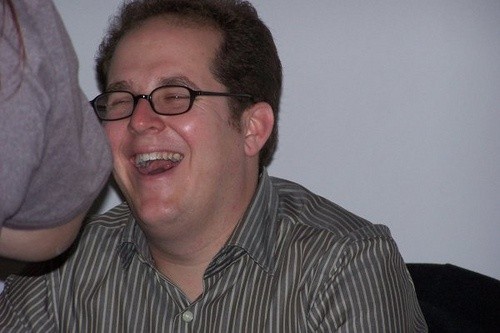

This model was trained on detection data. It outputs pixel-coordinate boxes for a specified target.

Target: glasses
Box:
[88,84,256,122]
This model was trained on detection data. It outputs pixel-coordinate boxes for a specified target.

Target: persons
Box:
[1,0,113,263]
[0,0,431,333]
